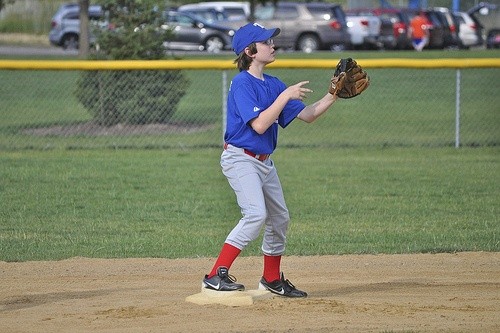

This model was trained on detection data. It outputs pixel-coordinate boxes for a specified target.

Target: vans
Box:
[178,1,250,17]
[214,2,349,53]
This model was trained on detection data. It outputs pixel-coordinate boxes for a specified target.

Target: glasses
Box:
[256,38,274,46]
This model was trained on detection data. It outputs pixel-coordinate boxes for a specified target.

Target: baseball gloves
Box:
[328,57,369,98]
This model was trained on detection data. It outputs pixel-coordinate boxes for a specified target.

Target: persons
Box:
[408,11,429,51]
[201,23,370,297]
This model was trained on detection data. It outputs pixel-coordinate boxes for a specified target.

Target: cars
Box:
[345,1,499,50]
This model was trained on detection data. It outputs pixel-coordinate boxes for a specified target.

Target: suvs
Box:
[49,4,108,50]
[105,9,231,54]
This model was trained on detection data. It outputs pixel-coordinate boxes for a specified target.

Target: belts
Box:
[224,142,270,161]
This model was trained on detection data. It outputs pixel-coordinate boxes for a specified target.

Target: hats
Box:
[231,21,281,55]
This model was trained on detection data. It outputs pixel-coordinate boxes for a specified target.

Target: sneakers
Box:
[260,272,308,298]
[202,266,246,291]
